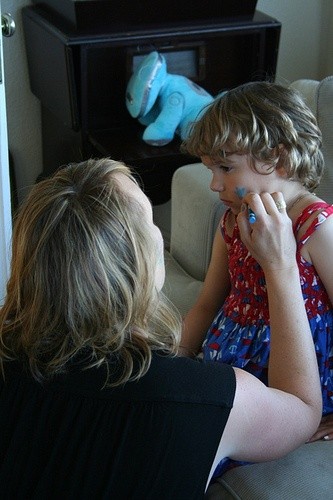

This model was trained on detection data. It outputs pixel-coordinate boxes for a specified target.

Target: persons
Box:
[0,159,322,499]
[173,81,333,420]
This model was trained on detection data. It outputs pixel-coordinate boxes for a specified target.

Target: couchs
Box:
[161,75,333,500]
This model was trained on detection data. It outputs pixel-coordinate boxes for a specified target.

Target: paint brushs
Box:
[247,204,256,224]
